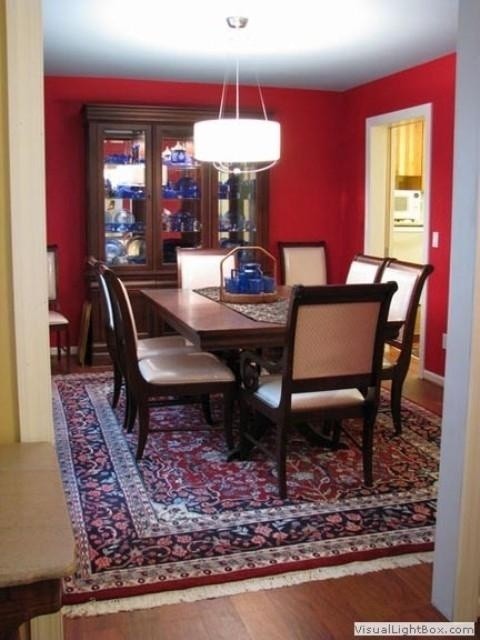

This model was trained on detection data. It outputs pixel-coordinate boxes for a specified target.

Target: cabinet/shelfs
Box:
[82,104,272,368]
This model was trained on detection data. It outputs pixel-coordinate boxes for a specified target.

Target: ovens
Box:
[391,189,423,227]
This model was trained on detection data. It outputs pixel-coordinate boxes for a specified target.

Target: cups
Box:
[231,264,263,293]
[224,276,274,295]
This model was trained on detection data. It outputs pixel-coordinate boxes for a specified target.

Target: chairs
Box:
[47,245,70,372]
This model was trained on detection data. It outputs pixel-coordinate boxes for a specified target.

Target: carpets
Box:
[50,369,442,619]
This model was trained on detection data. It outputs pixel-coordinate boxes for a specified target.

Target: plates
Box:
[104,239,127,264]
[110,209,136,232]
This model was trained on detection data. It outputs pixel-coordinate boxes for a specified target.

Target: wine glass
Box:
[122,223,136,232]
[109,223,121,233]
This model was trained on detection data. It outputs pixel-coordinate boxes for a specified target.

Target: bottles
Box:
[170,140,186,166]
[131,144,140,163]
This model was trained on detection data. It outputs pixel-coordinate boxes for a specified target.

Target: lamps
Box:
[192,12,282,175]
